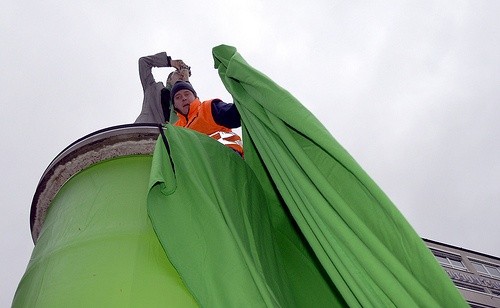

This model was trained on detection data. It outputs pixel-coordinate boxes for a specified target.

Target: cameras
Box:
[180,65,191,77]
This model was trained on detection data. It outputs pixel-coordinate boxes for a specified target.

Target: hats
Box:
[170,81,197,106]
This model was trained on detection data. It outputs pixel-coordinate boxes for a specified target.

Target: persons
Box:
[172,80,243,159]
[132,52,192,124]
